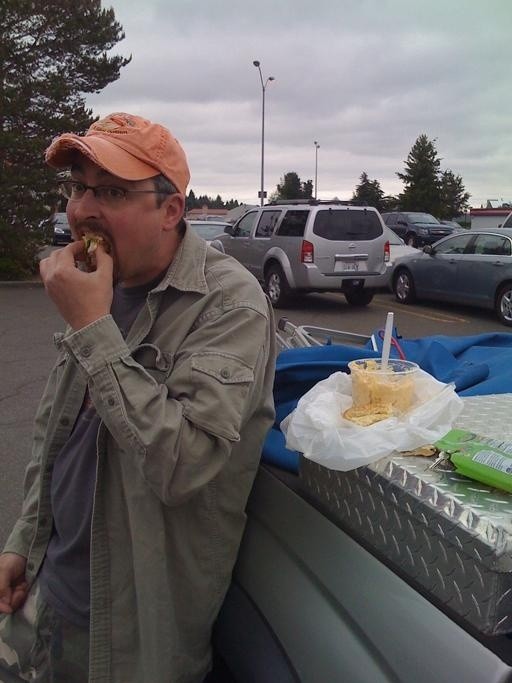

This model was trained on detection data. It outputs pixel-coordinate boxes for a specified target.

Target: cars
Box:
[43,211,74,246]
[187,217,232,245]
[388,228,511,327]
[384,226,424,264]
[440,220,467,234]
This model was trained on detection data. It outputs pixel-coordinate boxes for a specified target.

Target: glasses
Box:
[57,180,153,203]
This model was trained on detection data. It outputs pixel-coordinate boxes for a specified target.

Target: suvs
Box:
[380,210,455,248]
[208,200,394,309]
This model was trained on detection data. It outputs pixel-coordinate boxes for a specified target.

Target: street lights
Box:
[311,140,323,204]
[252,59,277,210]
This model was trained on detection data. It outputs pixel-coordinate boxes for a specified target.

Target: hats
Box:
[45,113,189,193]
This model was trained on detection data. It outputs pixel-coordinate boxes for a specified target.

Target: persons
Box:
[2,109,278,680]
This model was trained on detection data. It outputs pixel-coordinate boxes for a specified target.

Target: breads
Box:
[81,235,111,266]
[343,402,402,428]
